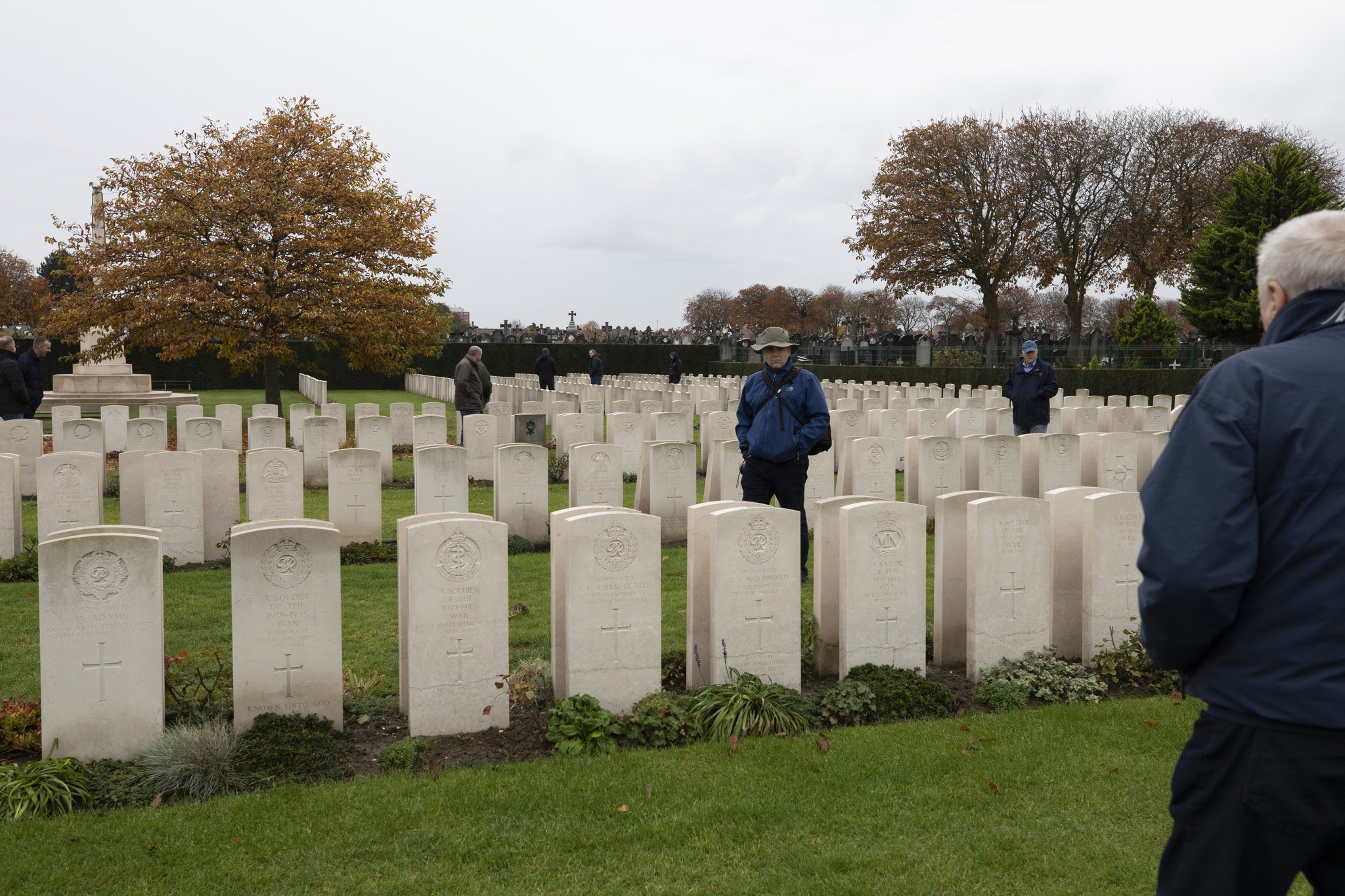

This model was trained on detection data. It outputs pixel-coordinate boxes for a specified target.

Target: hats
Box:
[750,327,800,355]
[542,348,549,354]
[1022,340,1037,354]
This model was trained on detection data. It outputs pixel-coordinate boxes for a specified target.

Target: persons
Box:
[1138,209,1345,895]
[18,336,51,418]
[1002,341,1058,437]
[454,346,492,447]
[588,349,603,386]
[669,352,684,384]
[533,348,559,390]
[735,327,830,583]
[0,335,29,421]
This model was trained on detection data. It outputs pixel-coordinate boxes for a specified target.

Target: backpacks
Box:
[751,364,832,456]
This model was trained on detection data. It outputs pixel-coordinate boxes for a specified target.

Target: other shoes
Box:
[801,572,809,585]
